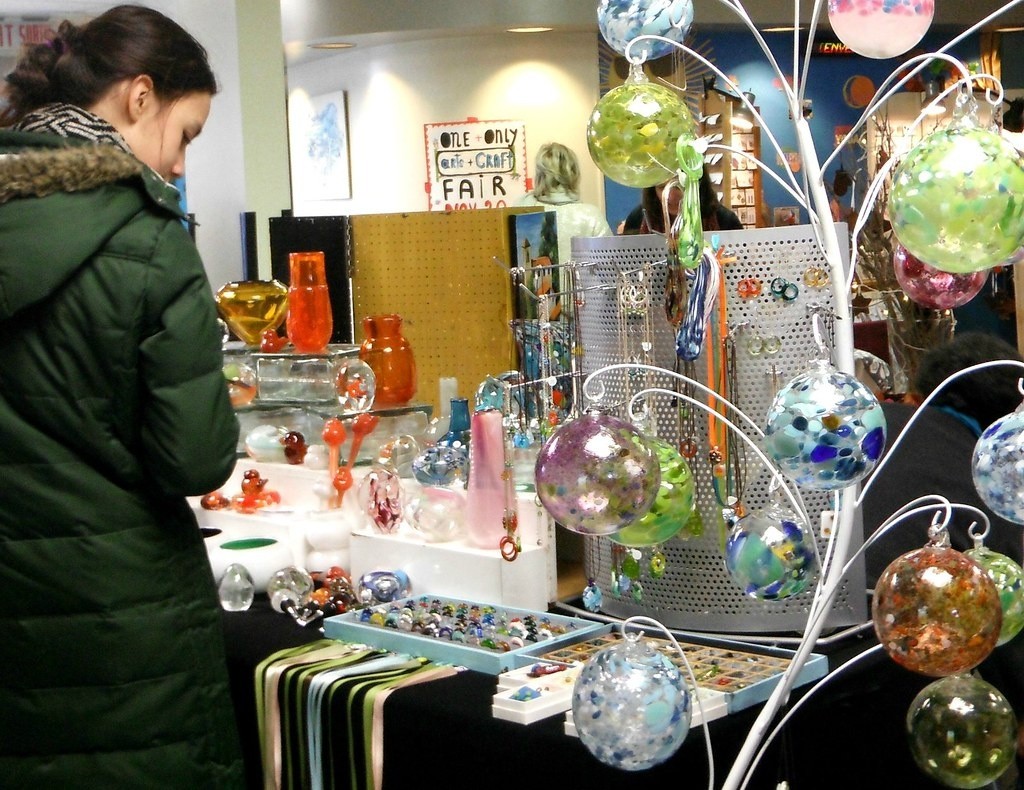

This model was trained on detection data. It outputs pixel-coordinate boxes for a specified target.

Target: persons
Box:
[623,163,742,234]
[0,8,248,790]
[861,333,1024,790]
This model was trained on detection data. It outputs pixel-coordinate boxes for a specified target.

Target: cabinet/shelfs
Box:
[706,91,763,231]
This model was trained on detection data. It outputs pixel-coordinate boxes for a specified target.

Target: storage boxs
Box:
[322,595,830,739]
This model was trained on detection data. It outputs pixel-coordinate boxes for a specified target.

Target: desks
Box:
[228,591,1024,790]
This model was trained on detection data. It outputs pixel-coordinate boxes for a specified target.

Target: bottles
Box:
[425,377,463,443]
[287,252,333,353]
[360,314,418,403]
[438,397,470,458]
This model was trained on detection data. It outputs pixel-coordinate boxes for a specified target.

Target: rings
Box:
[360,596,575,652]
